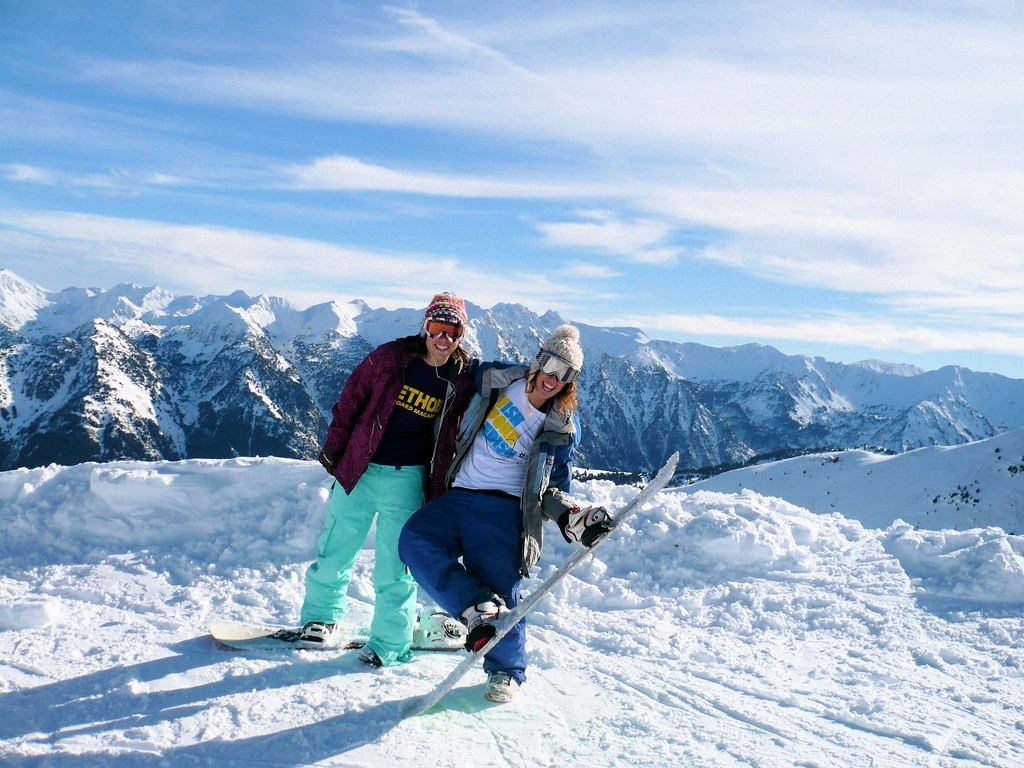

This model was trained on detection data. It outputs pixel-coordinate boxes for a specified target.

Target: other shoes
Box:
[300,621,336,649]
[356,642,388,668]
[485,670,523,705]
[460,600,506,651]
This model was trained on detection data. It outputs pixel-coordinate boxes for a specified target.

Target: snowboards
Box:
[201,615,473,656]
[393,451,681,724]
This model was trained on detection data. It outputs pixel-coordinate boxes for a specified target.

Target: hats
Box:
[421,294,466,343]
[528,323,584,383]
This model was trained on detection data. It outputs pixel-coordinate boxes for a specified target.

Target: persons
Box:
[298,292,477,666]
[397,324,612,703]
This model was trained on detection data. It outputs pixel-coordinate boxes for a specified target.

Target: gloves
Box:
[558,506,576,543]
[318,448,341,476]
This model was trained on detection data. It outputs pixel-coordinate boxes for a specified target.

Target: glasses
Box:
[536,347,581,384]
[423,317,464,345]
